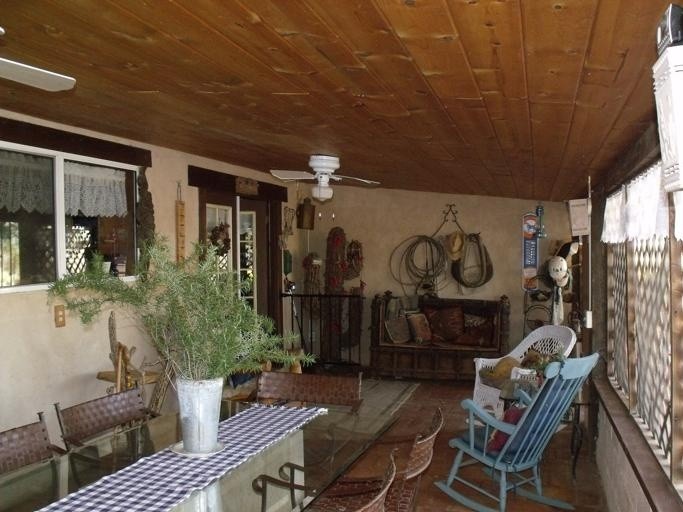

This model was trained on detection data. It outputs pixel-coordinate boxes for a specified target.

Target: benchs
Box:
[369,293,511,383]
[0,412,110,512]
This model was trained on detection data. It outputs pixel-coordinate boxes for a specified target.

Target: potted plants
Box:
[45,232,312,459]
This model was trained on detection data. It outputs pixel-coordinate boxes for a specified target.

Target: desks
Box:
[0,395,403,512]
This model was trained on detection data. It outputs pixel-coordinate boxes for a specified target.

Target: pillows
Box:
[385,306,494,347]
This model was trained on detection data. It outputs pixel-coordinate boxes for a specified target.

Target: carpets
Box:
[305,372,422,436]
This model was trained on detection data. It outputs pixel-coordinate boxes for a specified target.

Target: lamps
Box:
[311,175,334,202]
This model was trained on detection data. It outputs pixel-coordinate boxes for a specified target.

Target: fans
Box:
[269,155,381,185]
[0,27,77,93]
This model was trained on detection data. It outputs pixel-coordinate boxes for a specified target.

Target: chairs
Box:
[279,405,444,506]
[254,369,364,464]
[253,446,400,512]
[433,352,603,512]
[465,324,579,434]
[53,378,165,492]
